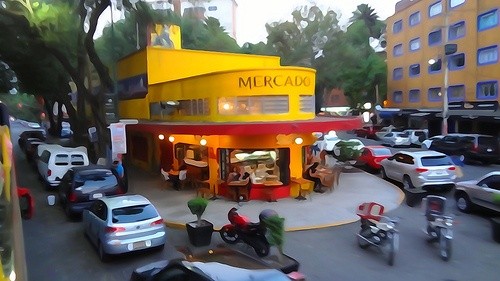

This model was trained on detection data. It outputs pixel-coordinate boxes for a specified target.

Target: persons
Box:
[110,160,125,188]
[160,161,325,202]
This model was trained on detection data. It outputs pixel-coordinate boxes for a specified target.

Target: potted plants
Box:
[262,216,299,275]
[186,198,214,246]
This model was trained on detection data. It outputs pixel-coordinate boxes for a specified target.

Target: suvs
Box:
[430,133,497,163]
[380,150,457,195]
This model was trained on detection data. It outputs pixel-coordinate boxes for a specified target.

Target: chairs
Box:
[301,181,314,201]
[332,167,341,186]
[179,170,188,181]
[195,182,210,198]
[161,168,170,181]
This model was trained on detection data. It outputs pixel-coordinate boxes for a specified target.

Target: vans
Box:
[36,142,90,186]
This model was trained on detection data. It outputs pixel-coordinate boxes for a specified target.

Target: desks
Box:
[228,181,249,204]
[170,170,181,191]
[292,178,308,201]
[315,171,334,193]
[263,181,284,202]
[203,179,225,201]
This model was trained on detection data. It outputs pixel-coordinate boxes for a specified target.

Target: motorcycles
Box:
[357,201,399,265]
[220,205,278,257]
[420,195,454,259]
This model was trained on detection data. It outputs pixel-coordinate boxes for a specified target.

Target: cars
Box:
[356,124,445,149]
[454,170,500,214]
[356,145,392,173]
[312,131,365,162]
[83,194,166,261]
[58,164,125,219]
